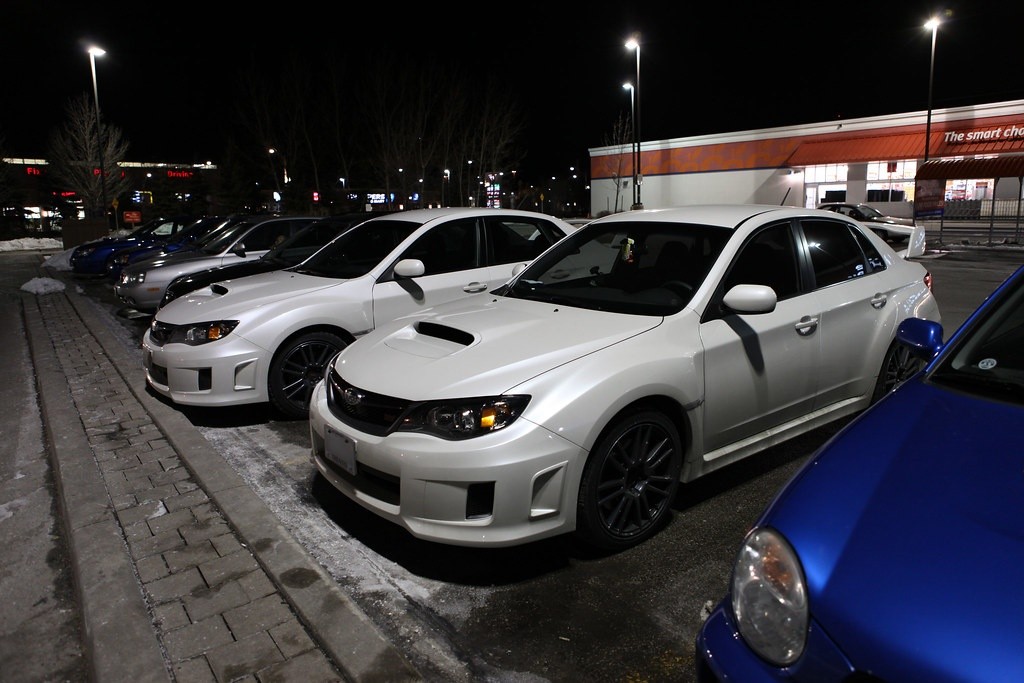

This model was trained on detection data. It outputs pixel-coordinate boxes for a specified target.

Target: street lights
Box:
[340,178,345,189]
[922,16,941,162]
[88,47,110,236]
[624,83,636,206]
[444,169,450,181]
[626,39,644,211]
[268,147,287,184]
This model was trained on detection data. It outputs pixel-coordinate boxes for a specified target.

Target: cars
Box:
[153,214,525,321]
[692,261,1023,683]
[142,207,621,421]
[68,204,346,317]
[816,202,915,242]
[309,205,940,560]
